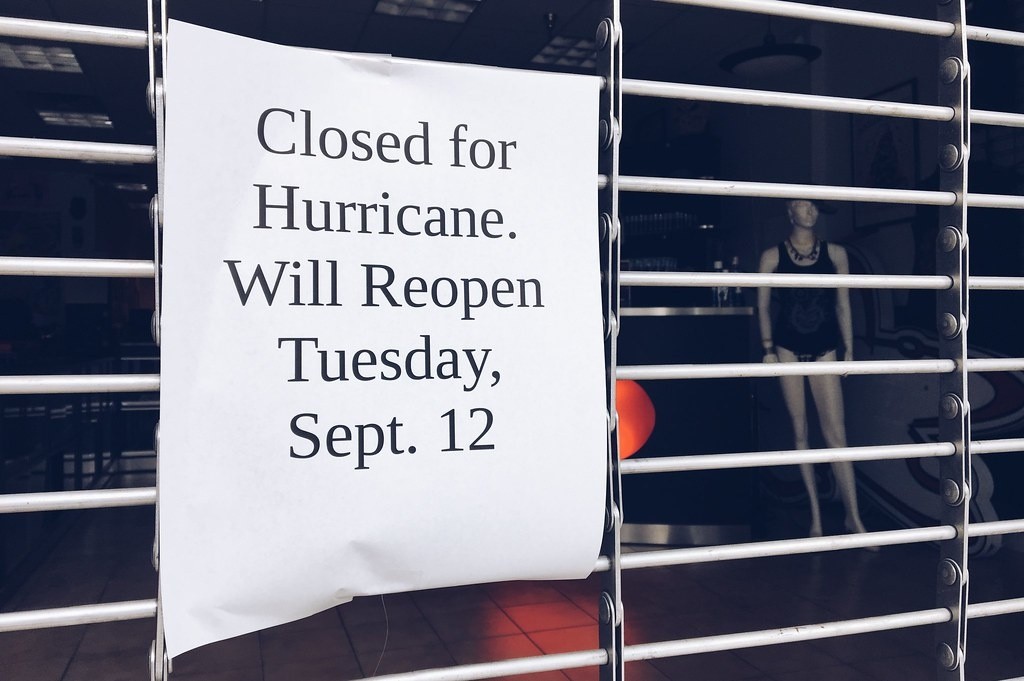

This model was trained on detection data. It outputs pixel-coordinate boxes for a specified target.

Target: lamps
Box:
[718,15,823,79]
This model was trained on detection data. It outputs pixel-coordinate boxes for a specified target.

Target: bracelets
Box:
[762,347,777,355]
[762,339,774,342]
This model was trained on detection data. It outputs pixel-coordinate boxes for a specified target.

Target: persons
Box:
[758,199,881,552]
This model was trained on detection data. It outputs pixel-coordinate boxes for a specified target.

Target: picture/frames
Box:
[849,77,921,232]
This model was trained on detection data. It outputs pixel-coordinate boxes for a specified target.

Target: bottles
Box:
[712,261,728,307]
[730,256,746,307]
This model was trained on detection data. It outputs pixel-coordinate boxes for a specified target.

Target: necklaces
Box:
[788,238,818,260]
